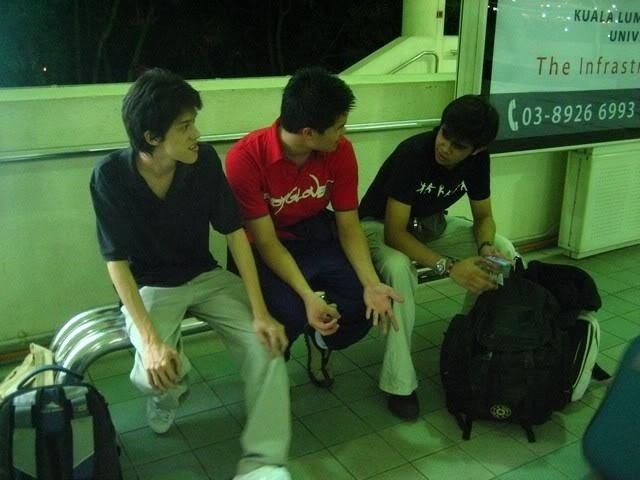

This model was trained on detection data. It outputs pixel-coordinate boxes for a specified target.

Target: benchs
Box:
[48,259,450,385]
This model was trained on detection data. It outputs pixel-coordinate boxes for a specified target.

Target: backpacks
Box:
[0,365,123,480]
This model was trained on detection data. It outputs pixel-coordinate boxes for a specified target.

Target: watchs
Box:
[433,253,451,277]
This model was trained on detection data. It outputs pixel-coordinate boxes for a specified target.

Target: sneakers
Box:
[388,391,419,419]
[147,400,174,434]
[304,324,334,387]
[232,465,292,480]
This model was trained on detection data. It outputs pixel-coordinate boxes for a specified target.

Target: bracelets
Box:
[474,241,493,254]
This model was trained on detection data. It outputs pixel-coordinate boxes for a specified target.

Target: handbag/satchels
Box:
[407,210,448,243]
[440,258,612,443]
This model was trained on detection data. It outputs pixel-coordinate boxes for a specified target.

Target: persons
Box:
[219,66,405,390]
[354,88,532,424]
[88,64,296,479]
